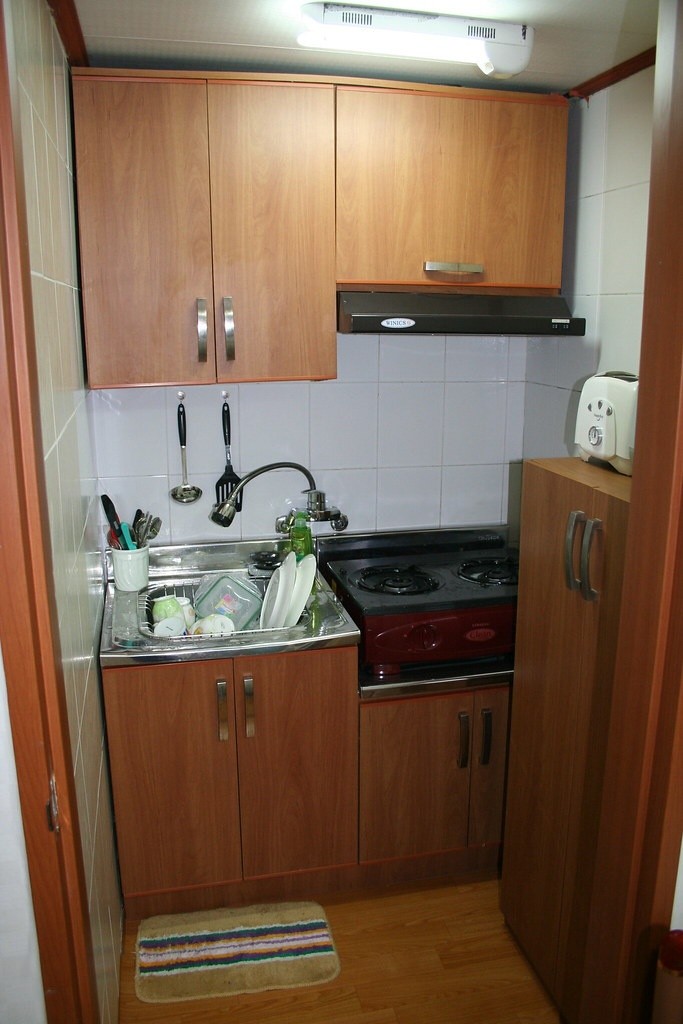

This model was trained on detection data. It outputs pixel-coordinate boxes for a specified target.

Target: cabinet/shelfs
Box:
[497,463,630,1024]
[360,682,512,900]
[71,76,337,390]
[336,85,569,295]
[100,644,359,917]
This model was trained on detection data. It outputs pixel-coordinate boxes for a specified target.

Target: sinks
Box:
[145,576,311,642]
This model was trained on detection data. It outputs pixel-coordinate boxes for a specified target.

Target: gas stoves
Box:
[319,530,519,675]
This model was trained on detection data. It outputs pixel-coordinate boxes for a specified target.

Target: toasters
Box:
[574,370,640,476]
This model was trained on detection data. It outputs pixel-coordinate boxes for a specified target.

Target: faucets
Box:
[208,461,326,527]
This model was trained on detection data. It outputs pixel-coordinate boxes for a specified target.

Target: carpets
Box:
[130,900,343,1005]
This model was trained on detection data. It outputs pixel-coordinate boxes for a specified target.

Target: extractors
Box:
[337,291,586,336]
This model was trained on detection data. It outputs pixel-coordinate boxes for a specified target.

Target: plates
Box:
[284,554,316,627]
[260,551,296,629]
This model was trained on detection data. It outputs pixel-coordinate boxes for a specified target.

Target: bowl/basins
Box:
[151,595,235,639]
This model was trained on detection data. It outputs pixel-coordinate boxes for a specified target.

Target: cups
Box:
[111,542,150,592]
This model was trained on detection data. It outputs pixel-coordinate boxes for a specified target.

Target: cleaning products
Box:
[288,511,313,562]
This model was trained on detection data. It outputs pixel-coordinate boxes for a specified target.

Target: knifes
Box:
[101,494,127,549]
